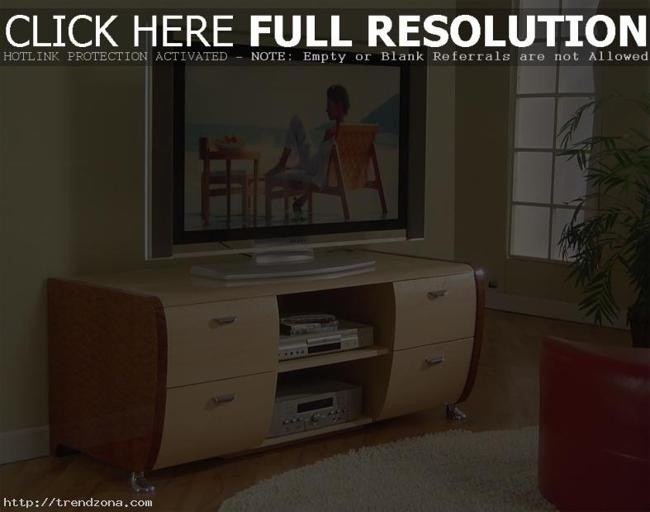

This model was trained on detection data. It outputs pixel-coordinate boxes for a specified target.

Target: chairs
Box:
[199,122,387,225]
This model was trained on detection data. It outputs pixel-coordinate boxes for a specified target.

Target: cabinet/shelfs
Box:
[48,248,486,494]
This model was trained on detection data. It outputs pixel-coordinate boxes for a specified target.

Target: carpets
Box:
[218,424,563,512]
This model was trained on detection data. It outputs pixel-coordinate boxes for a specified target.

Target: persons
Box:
[264,83,352,211]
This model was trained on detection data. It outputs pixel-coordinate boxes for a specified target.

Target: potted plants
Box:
[553,71,649,349]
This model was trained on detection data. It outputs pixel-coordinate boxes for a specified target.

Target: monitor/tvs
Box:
[144,22,430,280]
